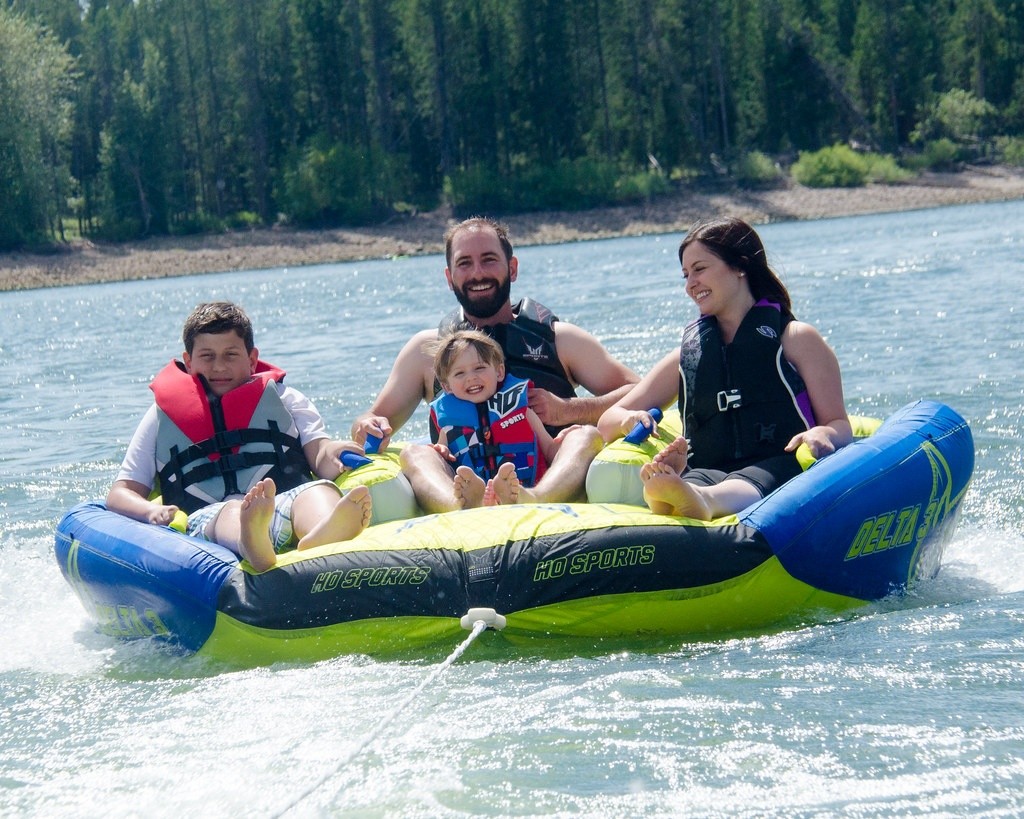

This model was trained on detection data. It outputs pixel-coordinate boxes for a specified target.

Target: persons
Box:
[106,302,370,574]
[594,217,853,522]
[348,218,643,515]
[429,332,582,508]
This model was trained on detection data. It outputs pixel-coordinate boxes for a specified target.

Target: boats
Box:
[57,402,977,667]
